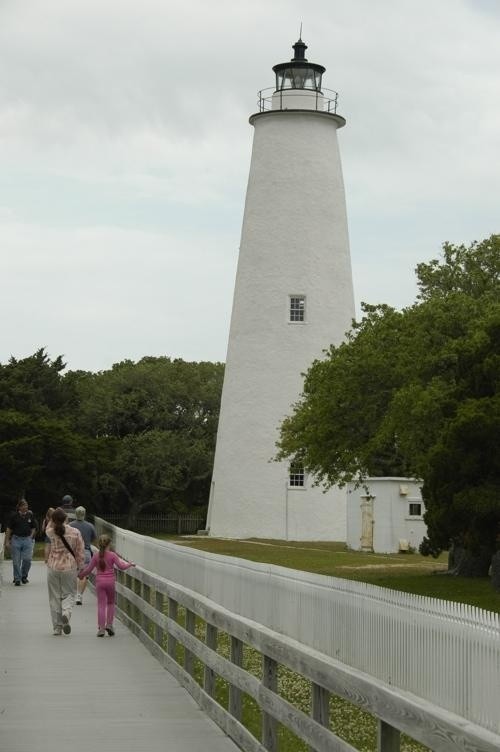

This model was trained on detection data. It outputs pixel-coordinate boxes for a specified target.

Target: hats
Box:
[63,494,73,502]
[76,506,86,521]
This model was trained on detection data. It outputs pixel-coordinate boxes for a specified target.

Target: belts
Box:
[14,533,32,538]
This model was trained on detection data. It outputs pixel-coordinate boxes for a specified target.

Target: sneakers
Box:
[62,615,72,635]
[21,576,29,584]
[106,624,116,637]
[53,627,62,636]
[15,579,21,586]
[76,594,83,606]
[96,627,105,638]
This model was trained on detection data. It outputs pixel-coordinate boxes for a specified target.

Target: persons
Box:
[45,506,83,635]
[28,509,39,556]
[4,498,37,585]
[68,505,97,604]
[41,507,57,564]
[57,494,76,524]
[79,534,138,637]
